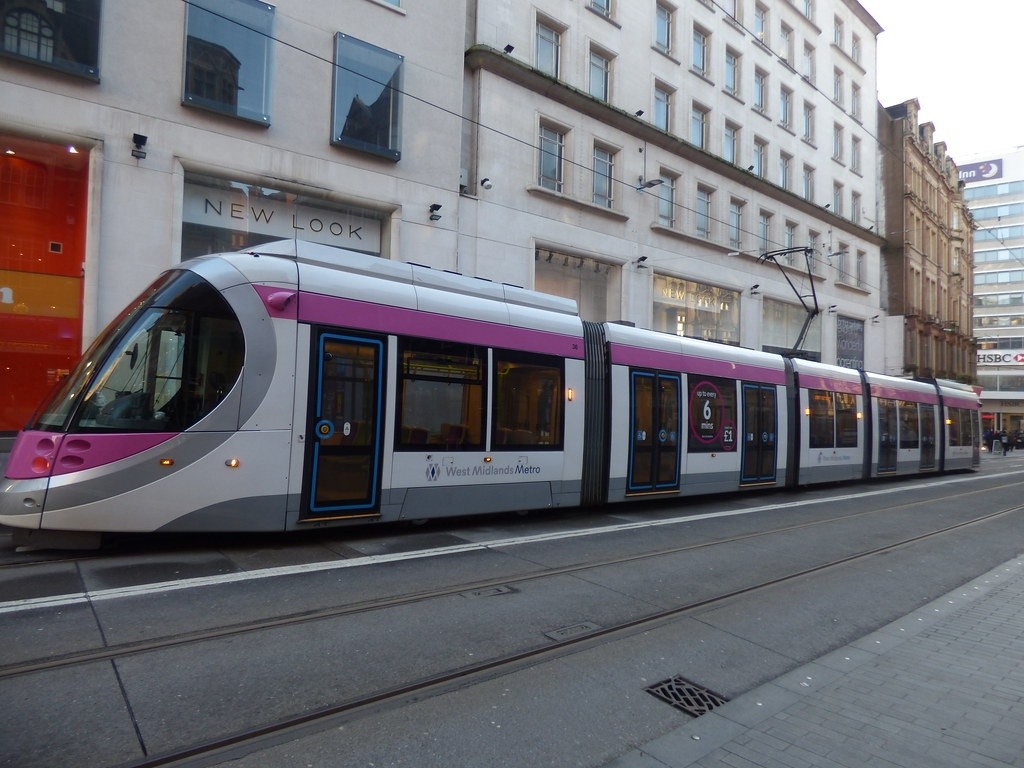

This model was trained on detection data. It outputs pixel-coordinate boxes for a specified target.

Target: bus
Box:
[0,239,981,554]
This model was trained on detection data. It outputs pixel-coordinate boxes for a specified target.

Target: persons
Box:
[984,428,1024,456]
